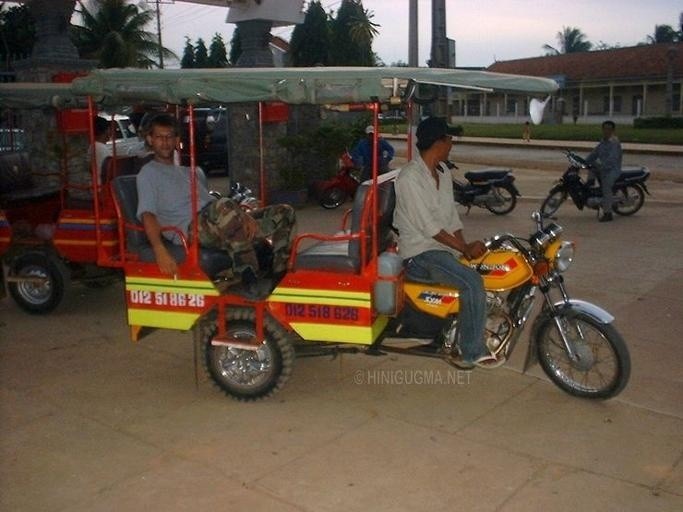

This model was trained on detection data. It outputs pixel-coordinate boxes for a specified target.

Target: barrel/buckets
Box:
[373,246,403,315]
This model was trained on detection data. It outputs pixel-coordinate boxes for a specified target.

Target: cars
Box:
[89,102,229,174]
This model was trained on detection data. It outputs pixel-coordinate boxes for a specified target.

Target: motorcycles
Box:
[317,144,363,211]
[446,159,522,217]
[539,146,654,222]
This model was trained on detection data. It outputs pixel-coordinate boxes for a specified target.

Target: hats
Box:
[416,117,464,139]
[365,125,375,134]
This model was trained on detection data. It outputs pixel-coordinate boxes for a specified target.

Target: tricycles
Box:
[69,65,632,402]
[0,80,262,315]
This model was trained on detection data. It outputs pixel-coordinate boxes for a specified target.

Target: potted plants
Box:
[272,172,308,202]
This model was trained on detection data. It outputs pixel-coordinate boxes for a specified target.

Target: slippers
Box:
[464,344,506,369]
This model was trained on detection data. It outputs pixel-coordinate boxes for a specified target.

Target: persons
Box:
[391,116,509,370]
[85,116,113,187]
[135,112,296,300]
[586,119,624,222]
[523,119,532,142]
[349,124,395,184]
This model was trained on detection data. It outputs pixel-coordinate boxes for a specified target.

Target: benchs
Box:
[114,175,232,272]
[0,151,59,208]
[288,168,401,274]
